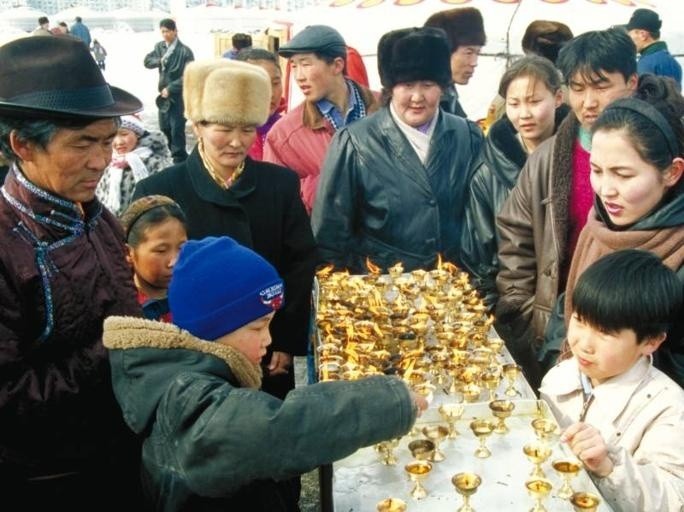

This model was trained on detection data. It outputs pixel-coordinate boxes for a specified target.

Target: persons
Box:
[0,8,684,512]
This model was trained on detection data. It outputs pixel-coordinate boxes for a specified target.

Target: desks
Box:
[314,275,610,511]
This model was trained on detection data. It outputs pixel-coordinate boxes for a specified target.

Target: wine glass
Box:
[489,400,514,433]
[314,266,524,402]
[526,480,552,512]
[379,438,402,465]
[438,404,465,438]
[470,420,495,458]
[450,473,481,512]
[404,460,432,498]
[378,498,407,510]
[523,445,552,479]
[532,417,557,444]
[422,426,449,462]
[570,493,600,512]
[410,441,435,461]
[552,458,580,498]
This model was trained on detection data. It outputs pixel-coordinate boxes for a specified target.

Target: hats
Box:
[376,26,452,89]
[181,57,272,127]
[118,112,145,137]
[0,32,145,121]
[424,7,486,47]
[153,95,172,115]
[277,24,348,60]
[614,8,661,32]
[167,235,286,342]
[230,32,252,50]
[522,19,572,62]
